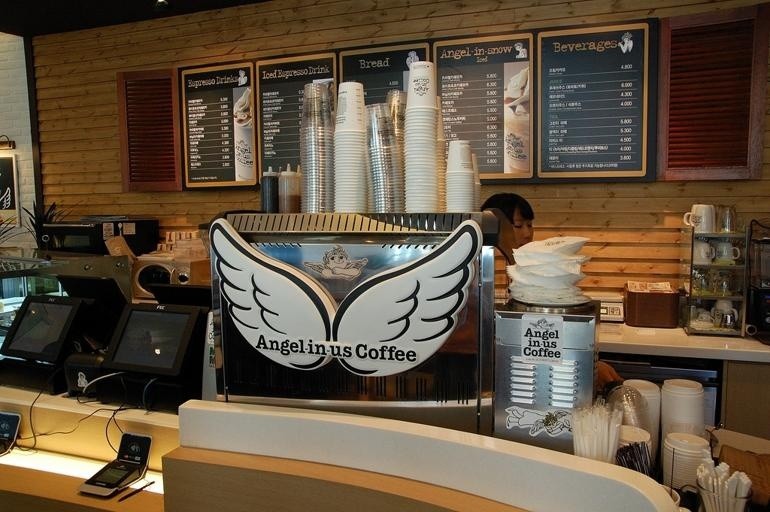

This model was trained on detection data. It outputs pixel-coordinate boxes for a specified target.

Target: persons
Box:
[480,192,626,391]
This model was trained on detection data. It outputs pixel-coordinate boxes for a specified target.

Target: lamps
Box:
[0,135,16,150]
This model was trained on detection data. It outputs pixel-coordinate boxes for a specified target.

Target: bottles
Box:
[260,164,299,214]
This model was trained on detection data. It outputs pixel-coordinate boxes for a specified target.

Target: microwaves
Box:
[130,253,213,304]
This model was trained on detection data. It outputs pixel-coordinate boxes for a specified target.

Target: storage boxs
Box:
[624,282,680,329]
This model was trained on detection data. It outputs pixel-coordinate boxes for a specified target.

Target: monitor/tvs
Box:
[0,295,82,364]
[101,303,201,378]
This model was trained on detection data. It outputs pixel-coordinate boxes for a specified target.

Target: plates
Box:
[662,432,712,494]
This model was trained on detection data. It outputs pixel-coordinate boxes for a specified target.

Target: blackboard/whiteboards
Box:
[255,49,338,191]
[178,59,256,191]
[339,38,430,105]
[431,28,535,186]
[535,17,660,184]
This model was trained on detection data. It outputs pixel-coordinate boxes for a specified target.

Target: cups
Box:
[300,60,481,213]
[682,202,742,331]
[696,479,754,512]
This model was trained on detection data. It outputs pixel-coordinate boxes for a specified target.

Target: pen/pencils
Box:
[118,481,155,502]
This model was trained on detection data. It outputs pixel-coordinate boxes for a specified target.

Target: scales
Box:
[582,291,624,321]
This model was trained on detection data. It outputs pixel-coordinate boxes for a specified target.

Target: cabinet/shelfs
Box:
[718,360,770,443]
[678,222,746,337]
